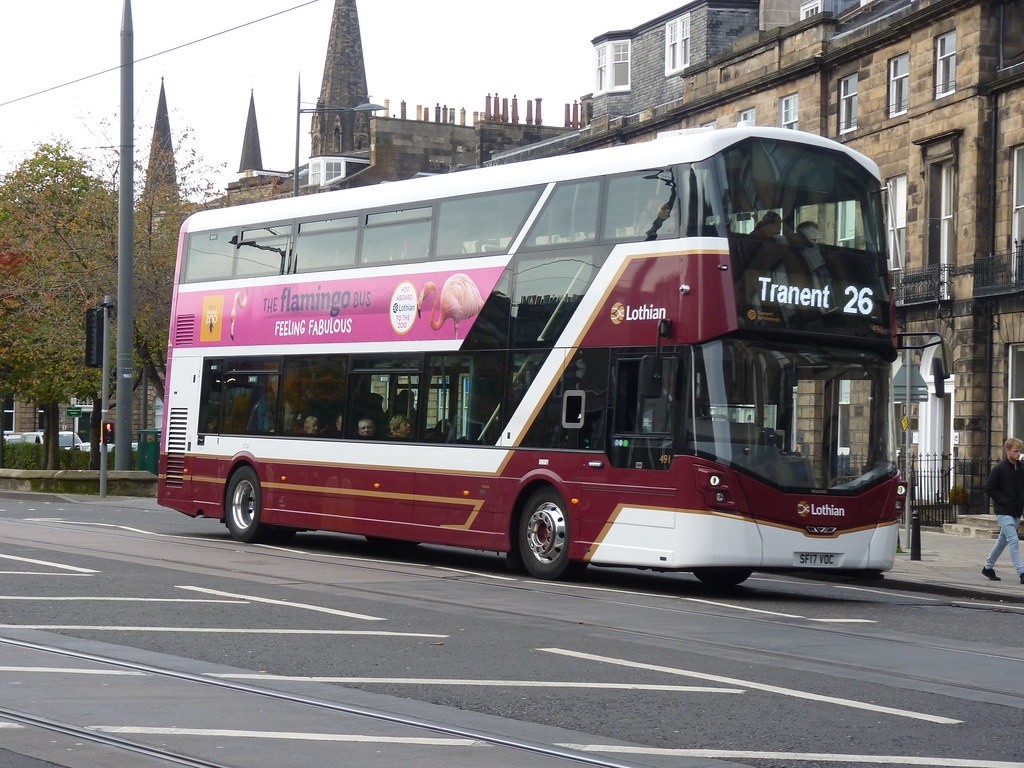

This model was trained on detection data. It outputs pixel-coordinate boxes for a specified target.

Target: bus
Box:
[157,121,951,583]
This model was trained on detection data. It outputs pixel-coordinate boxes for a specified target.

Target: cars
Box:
[2,430,136,452]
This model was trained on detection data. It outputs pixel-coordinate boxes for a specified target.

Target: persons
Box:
[981,438,1024,584]
[257,376,416,437]
[391,415,411,438]
[749,212,781,238]
[792,221,818,241]
[651,371,676,432]
[435,420,451,434]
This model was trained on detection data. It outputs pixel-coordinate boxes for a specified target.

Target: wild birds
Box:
[230,292,248,341]
[417,273,484,340]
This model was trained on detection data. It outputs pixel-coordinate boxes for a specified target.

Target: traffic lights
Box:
[104,423,115,443]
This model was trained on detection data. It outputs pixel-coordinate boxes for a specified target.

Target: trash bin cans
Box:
[136,428,162,475]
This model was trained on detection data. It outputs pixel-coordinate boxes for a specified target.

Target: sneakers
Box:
[1020,574,1024,583]
[981,567,1001,581]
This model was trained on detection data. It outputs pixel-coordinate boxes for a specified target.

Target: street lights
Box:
[293,73,388,197]
